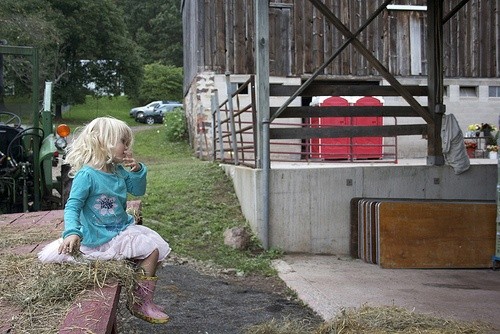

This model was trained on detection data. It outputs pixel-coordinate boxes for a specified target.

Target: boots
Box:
[126,272,169,324]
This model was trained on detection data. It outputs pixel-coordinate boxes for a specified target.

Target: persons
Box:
[36,117,172,325]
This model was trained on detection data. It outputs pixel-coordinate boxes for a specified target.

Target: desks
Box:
[0,200,143,334]
[464,136,492,158]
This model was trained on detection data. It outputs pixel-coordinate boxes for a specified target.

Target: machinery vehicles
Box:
[0,40,74,213]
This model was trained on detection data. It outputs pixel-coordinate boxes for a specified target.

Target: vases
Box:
[488,152,497,159]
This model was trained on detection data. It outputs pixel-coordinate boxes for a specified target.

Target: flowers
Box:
[486,145,498,152]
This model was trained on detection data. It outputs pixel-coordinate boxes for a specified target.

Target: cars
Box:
[129,99,183,126]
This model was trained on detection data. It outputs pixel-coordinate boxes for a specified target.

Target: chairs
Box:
[0,126,23,169]
